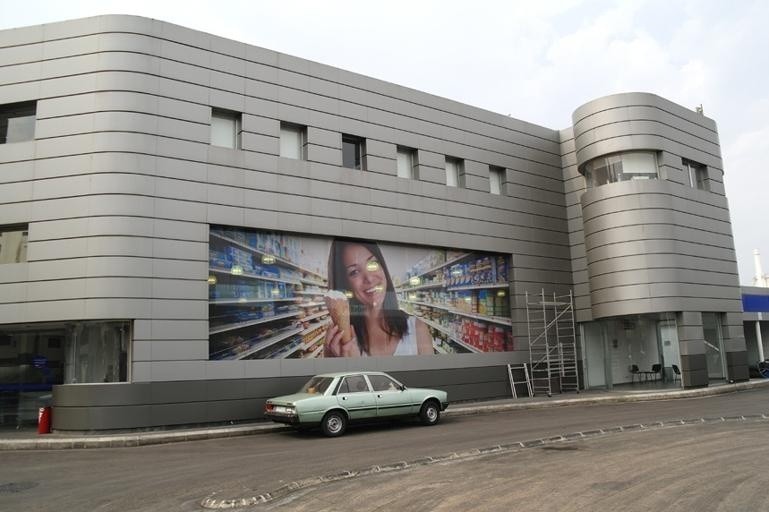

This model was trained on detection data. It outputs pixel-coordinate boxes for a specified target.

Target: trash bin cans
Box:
[38,406,51,434]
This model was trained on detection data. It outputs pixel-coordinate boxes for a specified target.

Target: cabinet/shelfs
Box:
[209,232,334,360]
[394,251,513,355]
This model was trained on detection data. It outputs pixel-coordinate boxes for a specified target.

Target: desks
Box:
[637,371,655,384]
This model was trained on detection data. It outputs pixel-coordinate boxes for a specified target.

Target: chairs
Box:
[673,365,681,384]
[630,364,641,384]
[652,364,662,382]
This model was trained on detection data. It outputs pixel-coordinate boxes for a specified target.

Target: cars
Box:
[264,371,449,437]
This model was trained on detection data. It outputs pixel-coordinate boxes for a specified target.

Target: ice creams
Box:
[323,289,352,344]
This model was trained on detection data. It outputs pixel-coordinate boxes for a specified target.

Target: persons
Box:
[322,237,435,358]
